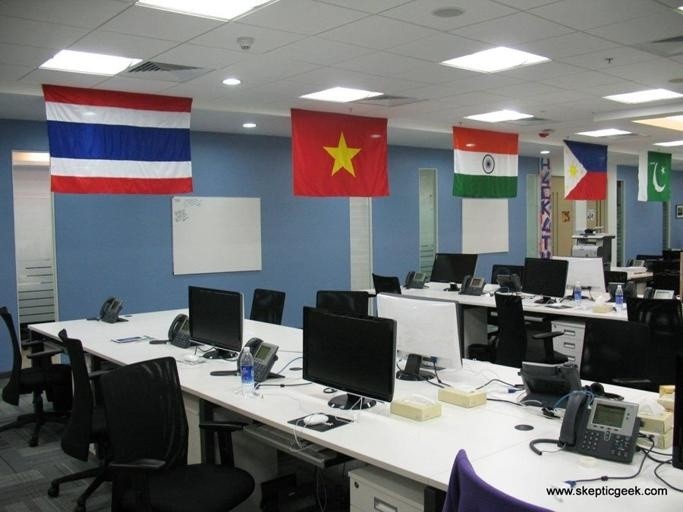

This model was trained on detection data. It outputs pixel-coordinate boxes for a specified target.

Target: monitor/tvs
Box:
[521,257,569,304]
[302,306,396,411]
[552,256,606,298]
[188,285,244,361]
[376,293,464,381]
[430,253,479,292]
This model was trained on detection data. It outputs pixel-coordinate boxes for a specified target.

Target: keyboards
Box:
[256,424,326,453]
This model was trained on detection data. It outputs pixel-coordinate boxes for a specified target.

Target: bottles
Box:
[575,282,581,306]
[240,346,254,400]
[615,285,623,312]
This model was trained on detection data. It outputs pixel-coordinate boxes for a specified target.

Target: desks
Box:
[27,308,682,512]
[375,282,631,359]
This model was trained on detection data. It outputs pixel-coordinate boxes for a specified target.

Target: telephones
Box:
[150,312,190,349]
[520,359,583,408]
[403,271,428,289]
[86,295,124,324]
[210,337,279,382]
[608,281,637,299]
[626,258,645,267]
[458,275,487,296]
[496,274,521,292]
[643,287,676,299]
[530,392,640,465]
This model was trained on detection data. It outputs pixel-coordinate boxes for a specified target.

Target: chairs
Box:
[372,273,401,295]
[494,292,568,368]
[249,288,286,325]
[91,355,257,512]
[47,328,125,512]
[315,290,368,316]
[491,264,524,285]
[0,306,70,449]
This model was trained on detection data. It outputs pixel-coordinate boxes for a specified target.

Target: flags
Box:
[42,82,194,194]
[291,108,390,196]
[452,125,519,198]
[564,139,608,200]
[637,149,672,202]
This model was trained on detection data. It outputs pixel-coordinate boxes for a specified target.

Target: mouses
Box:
[303,414,328,425]
[182,353,198,361]
